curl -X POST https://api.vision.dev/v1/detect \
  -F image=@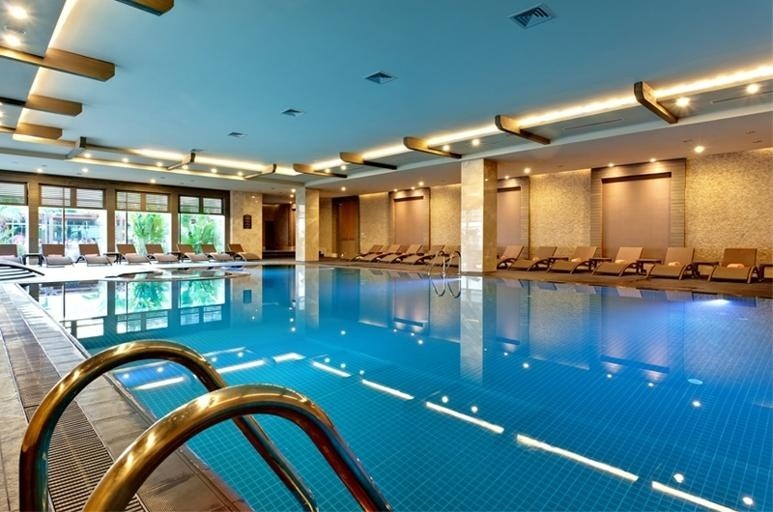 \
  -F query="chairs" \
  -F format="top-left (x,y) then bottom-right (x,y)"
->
top-left (0, 242), bottom-right (257, 273)
top-left (350, 240), bottom-right (461, 270)
top-left (592, 246), bottom-right (646, 280)
top-left (546, 245), bottom-right (601, 279)
top-left (511, 246), bottom-right (557, 272)
top-left (645, 247), bottom-right (698, 281)
top-left (707, 246), bottom-right (759, 284)
top-left (495, 243), bottom-right (524, 270)
